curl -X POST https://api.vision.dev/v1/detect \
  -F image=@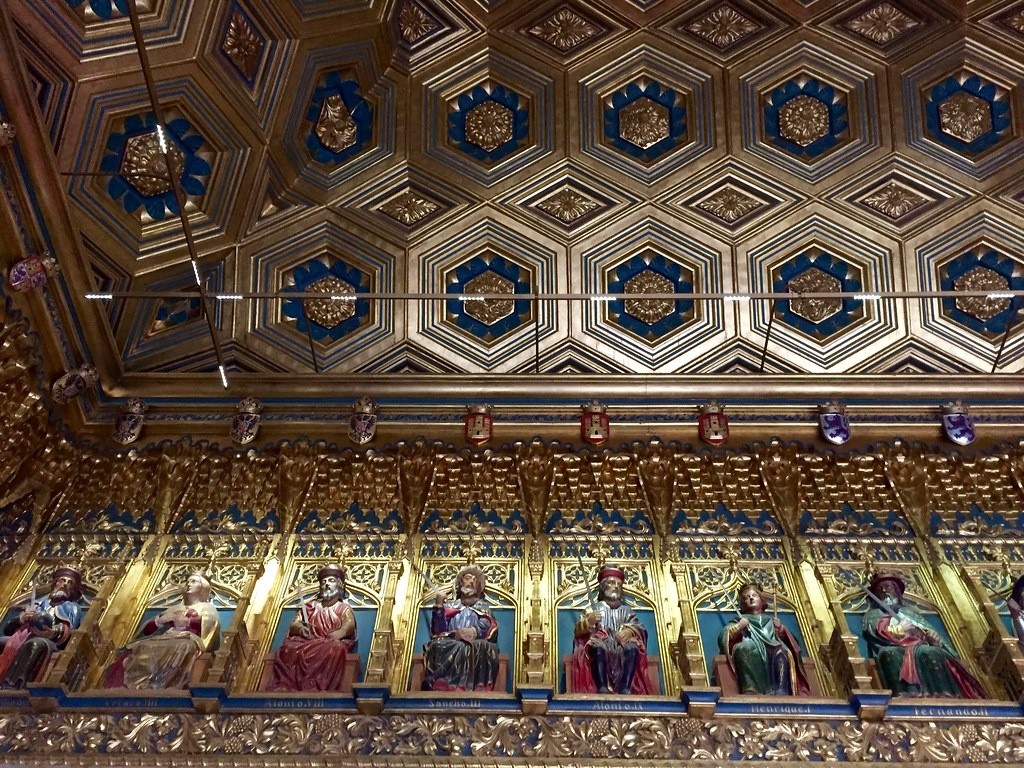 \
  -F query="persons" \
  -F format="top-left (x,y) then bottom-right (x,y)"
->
top-left (862, 570), bottom-right (987, 700)
top-left (718, 583), bottom-right (810, 697)
top-left (101, 573), bottom-right (219, 691)
top-left (273, 564), bottom-right (357, 692)
top-left (423, 565), bottom-right (501, 691)
top-left (1006, 574), bottom-right (1024, 647)
top-left (0, 566), bottom-right (83, 691)
top-left (571, 564), bottom-right (653, 694)
